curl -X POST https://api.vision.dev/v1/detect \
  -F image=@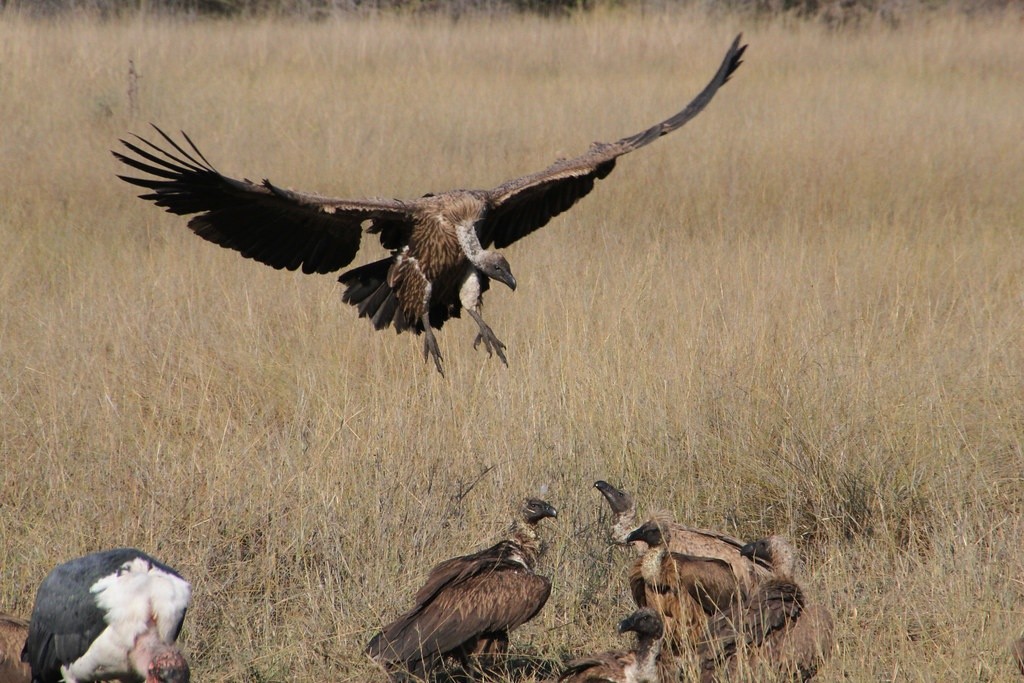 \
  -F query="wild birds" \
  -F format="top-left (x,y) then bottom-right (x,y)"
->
top-left (560, 607), bottom-right (665, 682)
top-left (363, 493), bottom-right (559, 682)
top-left (592, 479), bottom-right (835, 682)
top-left (106, 30), bottom-right (753, 380)
top-left (20, 546), bottom-right (195, 682)
